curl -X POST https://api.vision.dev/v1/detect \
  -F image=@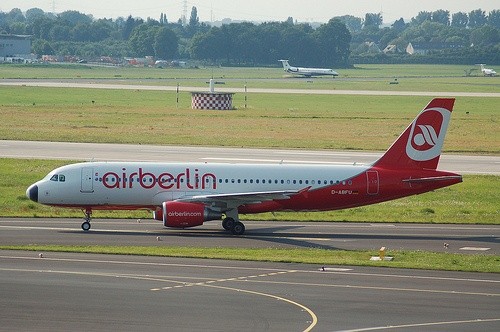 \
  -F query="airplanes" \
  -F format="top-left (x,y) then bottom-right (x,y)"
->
top-left (476, 64), bottom-right (497, 76)
top-left (280, 59), bottom-right (338, 79)
top-left (25, 97), bottom-right (463, 236)
